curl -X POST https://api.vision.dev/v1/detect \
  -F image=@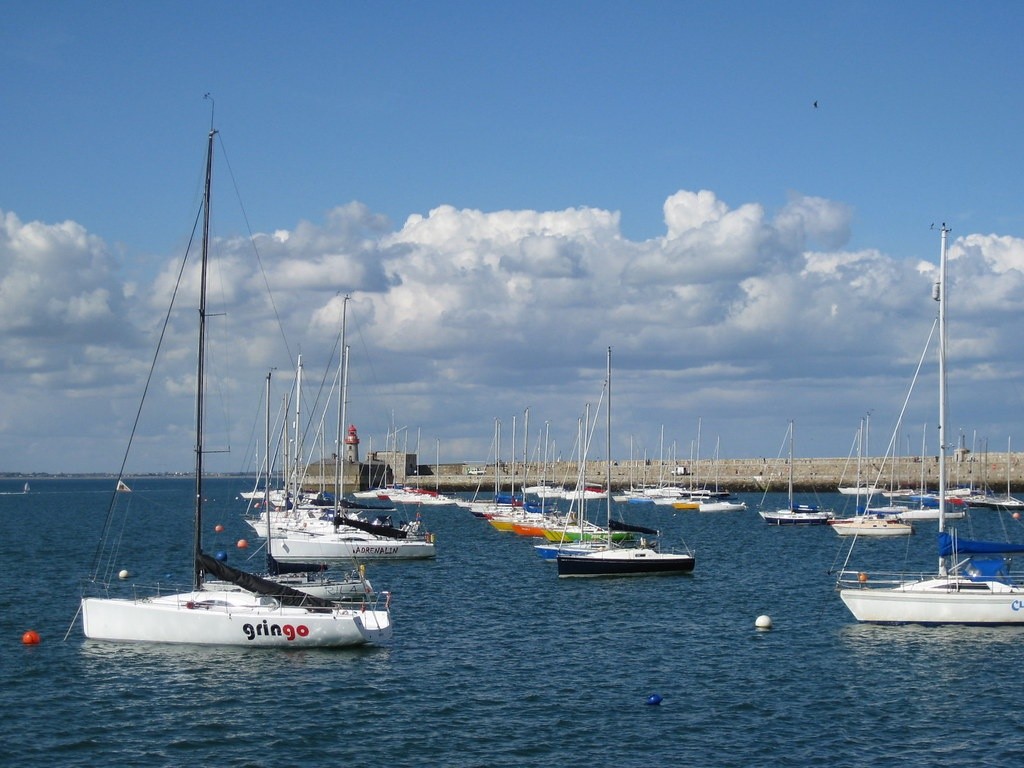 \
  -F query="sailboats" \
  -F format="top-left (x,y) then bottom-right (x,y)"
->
top-left (523, 412), bottom-right (1024, 536)
top-left (353, 394), bottom-right (694, 576)
top-left (240, 296), bottom-right (441, 566)
top-left (834, 223), bottom-right (1024, 622)
top-left (80, 125), bottom-right (390, 648)
